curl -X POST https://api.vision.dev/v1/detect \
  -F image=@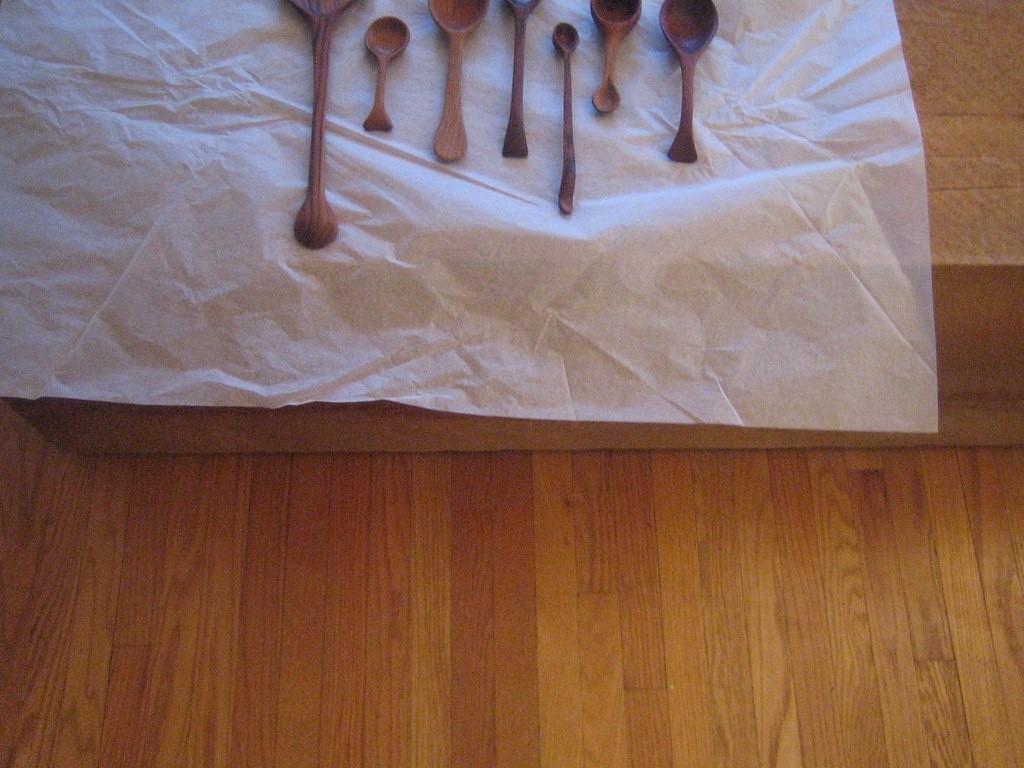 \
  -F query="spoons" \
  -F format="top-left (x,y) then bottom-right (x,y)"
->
top-left (590, 0), bottom-right (643, 113)
top-left (502, 0), bottom-right (541, 158)
top-left (552, 23), bottom-right (581, 215)
top-left (659, 0), bottom-right (719, 163)
top-left (363, 16), bottom-right (411, 132)
top-left (428, 0), bottom-right (490, 162)
